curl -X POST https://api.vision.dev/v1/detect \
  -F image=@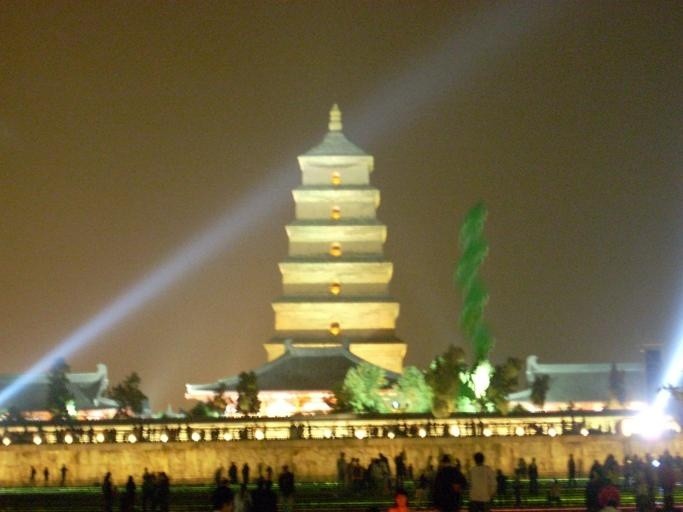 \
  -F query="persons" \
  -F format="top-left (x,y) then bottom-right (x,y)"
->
top-left (103, 469), bottom-right (170, 512)
top-left (337, 451), bottom-right (434, 508)
top-left (210, 462), bottom-right (295, 512)
top-left (31, 465), bottom-right (67, 483)
top-left (495, 454), bottom-right (683, 512)
top-left (429, 455), bottom-right (468, 512)
top-left (465, 453), bottom-right (497, 512)
